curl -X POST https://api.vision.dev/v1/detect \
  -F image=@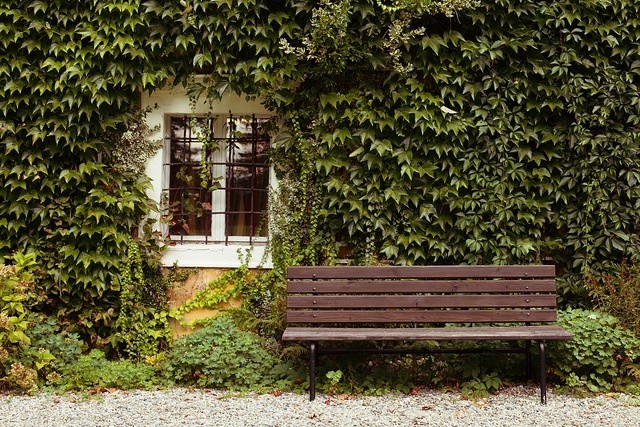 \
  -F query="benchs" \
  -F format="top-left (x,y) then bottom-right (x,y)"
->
top-left (280, 265), bottom-right (573, 405)
